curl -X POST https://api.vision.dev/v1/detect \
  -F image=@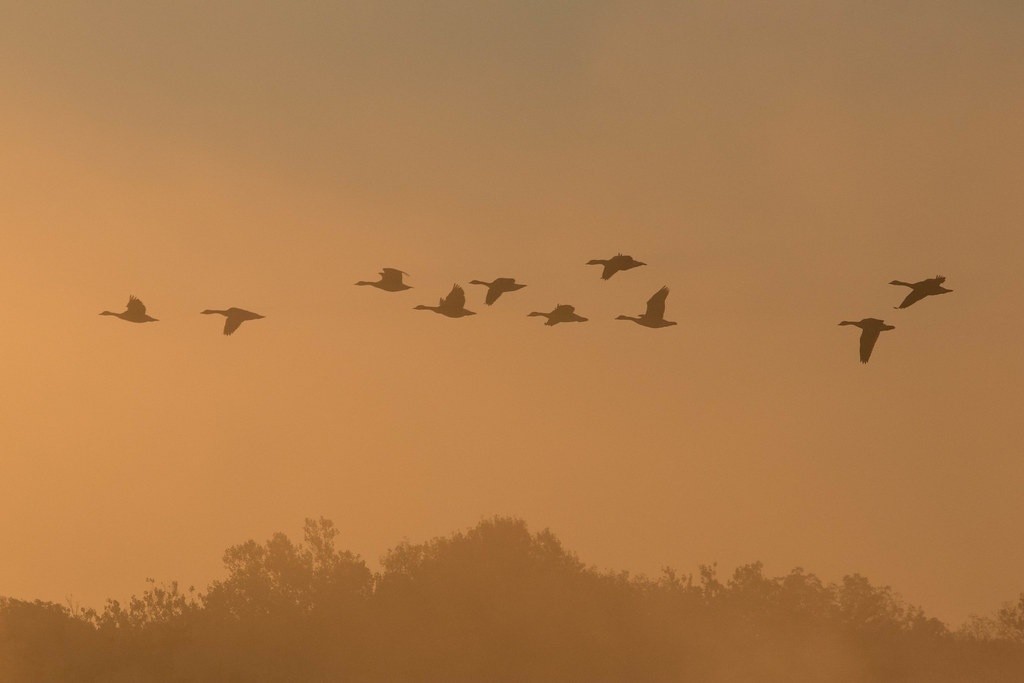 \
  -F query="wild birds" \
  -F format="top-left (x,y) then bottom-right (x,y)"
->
top-left (584, 253), bottom-right (647, 280)
top-left (97, 294), bottom-right (160, 323)
top-left (199, 307), bottom-right (266, 336)
top-left (353, 268), bottom-right (415, 292)
top-left (837, 318), bottom-right (896, 364)
top-left (526, 303), bottom-right (589, 328)
top-left (888, 274), bottom-right (954, 308)
top-left (411, 283), bottom-right (477, 318)
top-left (467, 277), bottom-right (528, 307)
top-left (614, 285), bottom-right (677, 328)
